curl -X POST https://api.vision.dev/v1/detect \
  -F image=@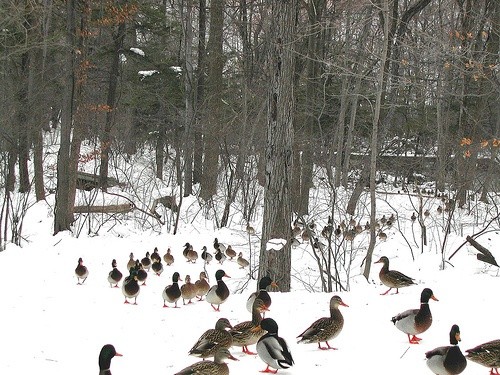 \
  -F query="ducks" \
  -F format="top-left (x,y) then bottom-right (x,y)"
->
top-left (424, 324), bottom-right (467, 375)
top-left (296, 296), bottom-right (349, 350)
top-left (75, 185), bottom-right (455, 312)
top-left (227, 299), bottom-right (270, 355)
top-left (173, 348), bottom-right (238, 375)
top-left (96, 343), bottom-right (123, 375)
top-left (465, 339), bottom-right (500, 375)
top-left (188, 318), bottom-right (239, 362)
top-left (391, 288), bottom-right (439, 344)
top-left (251, 318), bottom-right (295, 374)
